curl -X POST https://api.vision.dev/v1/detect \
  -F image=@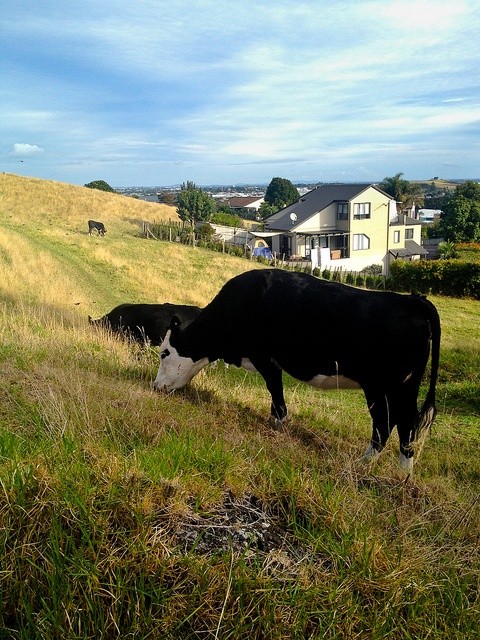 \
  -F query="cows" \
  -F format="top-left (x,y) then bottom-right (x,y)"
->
top-left (88, 220), bottom-right (107, 237)
top-left (152, 267), bottom-right (441, 479)
top-left (88, 303), bottom-right (202, 349)
